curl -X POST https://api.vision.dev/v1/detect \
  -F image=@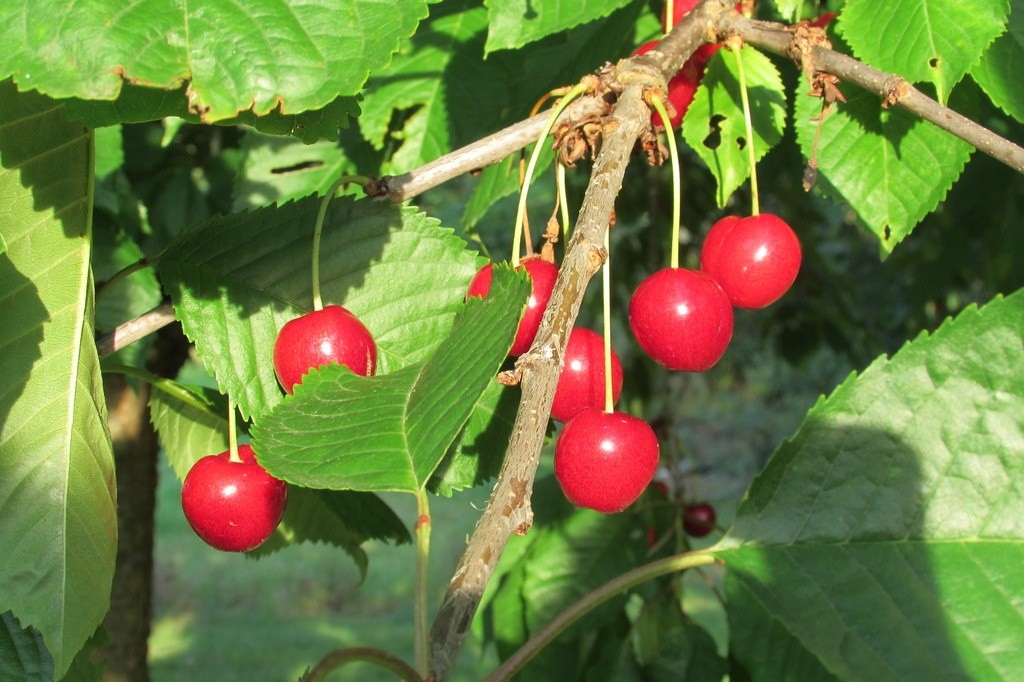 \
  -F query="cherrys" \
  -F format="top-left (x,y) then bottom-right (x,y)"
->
top-left (181, 0), bottom-right (838, 550)
